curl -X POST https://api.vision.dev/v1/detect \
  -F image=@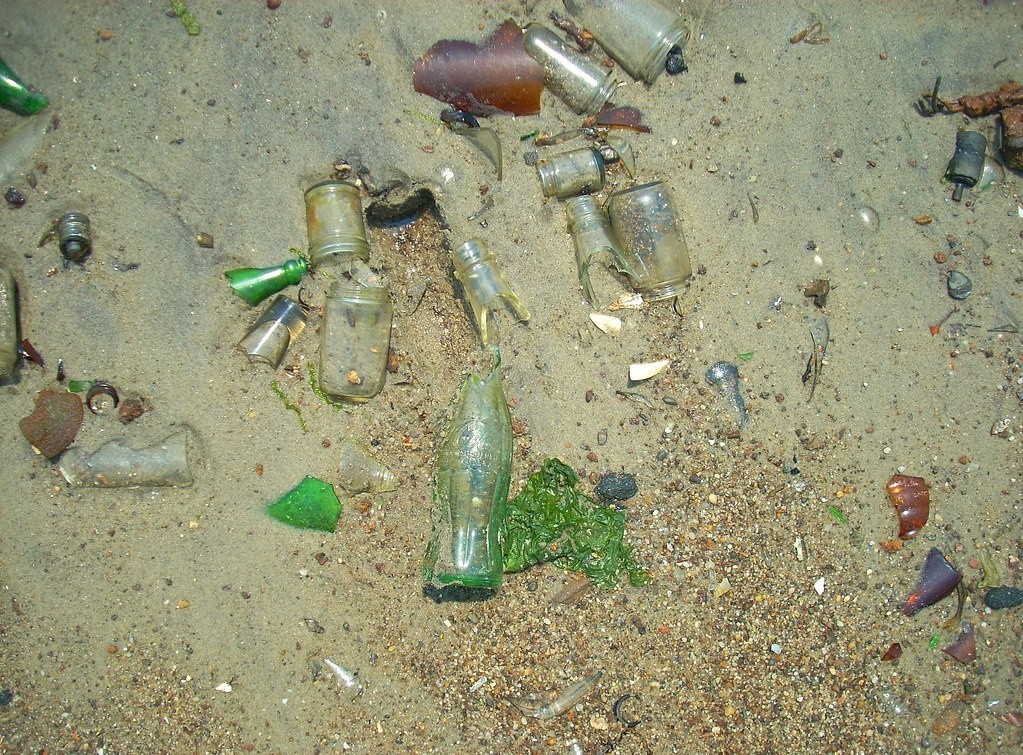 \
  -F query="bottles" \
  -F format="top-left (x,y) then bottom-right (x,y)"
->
top-left (304, 179), bottom-right (371, 267)
top-left (522, 21), bottom-right (619, 119)
top-left (608, 179), bottom-right (694, 302)
top-left (562, 1), bottom-right (691, 85)
top-left (318, 281), bottom-right (394, 403)
top-left (535, 146), bottom-right (606, 201)
top-left (422, 345), bottom-right (514, 602)
